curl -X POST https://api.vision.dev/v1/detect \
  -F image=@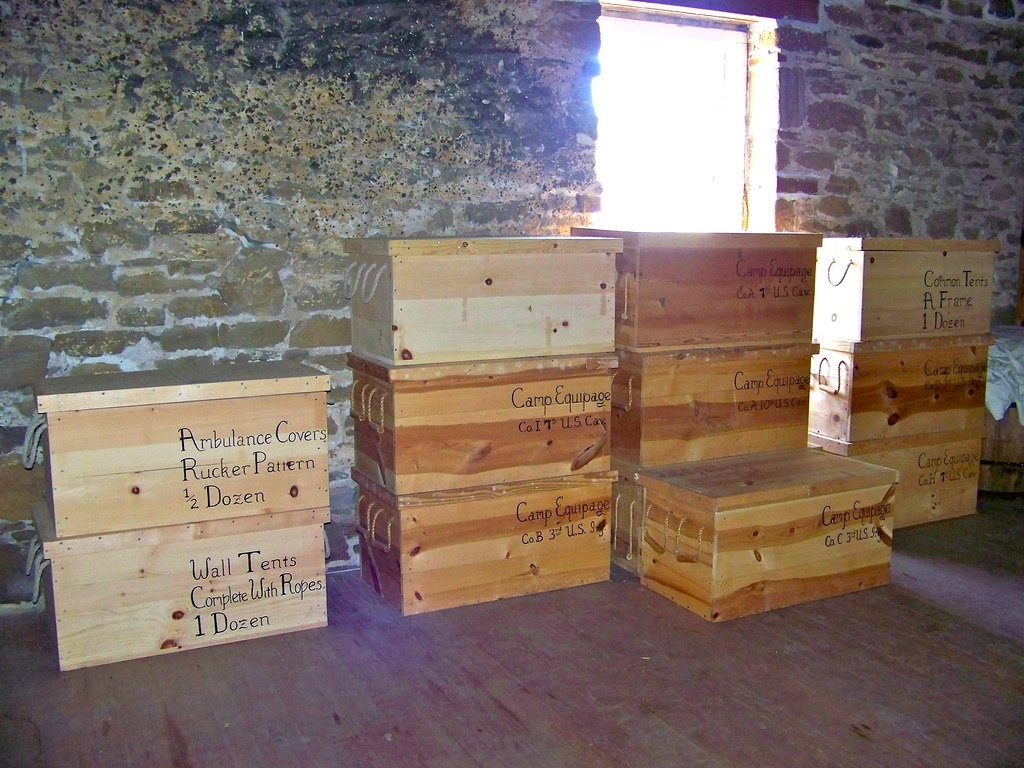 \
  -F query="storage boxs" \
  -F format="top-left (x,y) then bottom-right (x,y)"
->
top-left (340, 352), bottom-right (621, 500)
top-left (31, 357), bottom-right (331, 538)
top-left (350, 467), bottom-right (614, 618)
top-left (593, 442), bottom-right (824, 577)
top-left (633, 445), bottom-right (903, 623)
top-left (338, 234), bottom-right (626, 365)
top-left (806, 428), bottom-right (991, 530)
top-left (610, 343), bottom-right (821, 468)
top-left (569, 226), bottom-right (825, 352)
top-left (808, 236), bottom-right (1000, 339)
top-left (29, 509), bottom-right (329, 671)
top-left (808, 336), bottom-right (995, 443)
top-left (978, 403), bottom-right (1024, 491)
